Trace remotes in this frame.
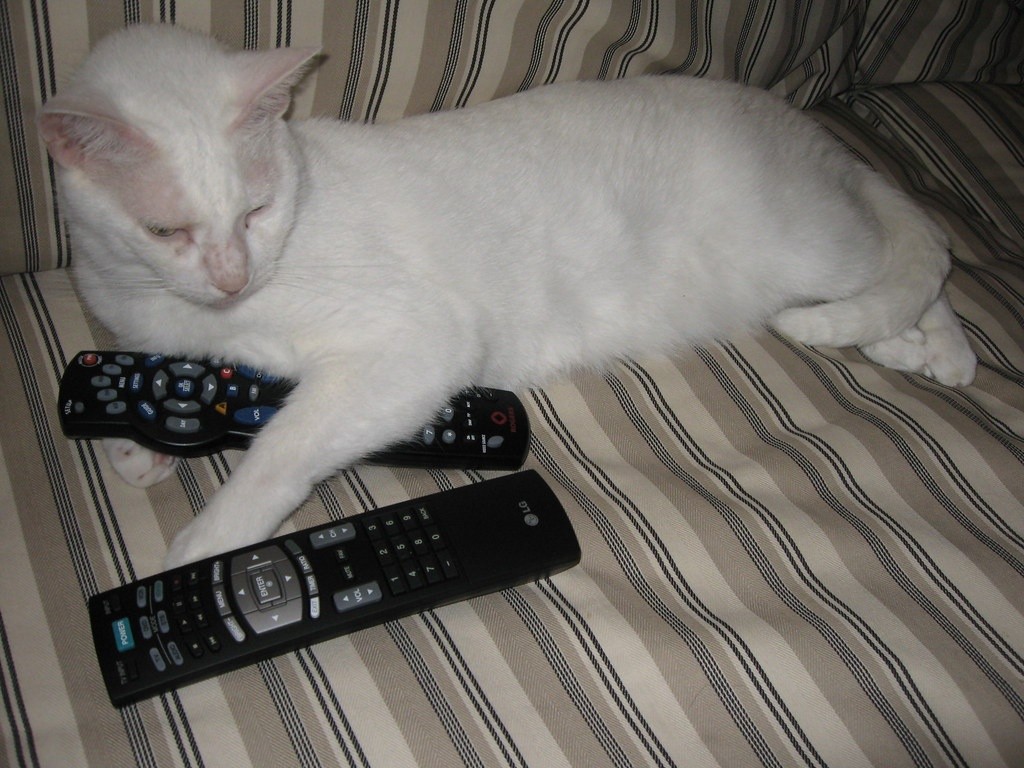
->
[55,350,532,471]
[87,469,581,708]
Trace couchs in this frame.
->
[0,0,1024,768]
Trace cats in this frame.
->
[31,15,976,569]
